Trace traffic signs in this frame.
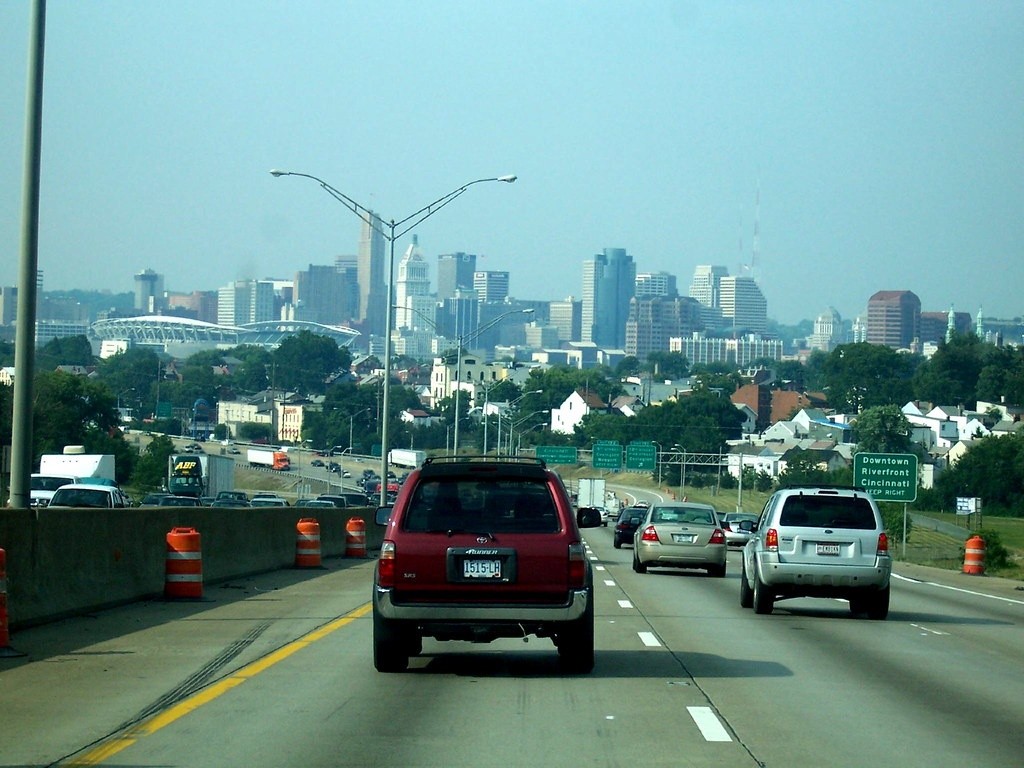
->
[593,440,657,472]
[535,446,578,464]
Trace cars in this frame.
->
[139,491,399,509]
[184,444,204,454]
[587,500,759,576]
[356,469,410,491]
[310,459,351,478]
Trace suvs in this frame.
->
[740,484,891,619]
[372,456,595,674]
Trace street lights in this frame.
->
[651,440,662,489]
[118,387,135,410]
[327,445,353,494]
[296,440,313,496]
[671,443,686,501]
[390,304,550,463]
[270,168,518,514]
[349,408,373,457]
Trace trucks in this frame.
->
[28,446,115,508]
[246,449,290,470]
[577,477,606,511]
[168,453,234,499]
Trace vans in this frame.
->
[45,484,125,509]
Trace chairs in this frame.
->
[222,495,229,500]
[237,496,243,499]
[786,510,809,526]
[417,493,454,513]
